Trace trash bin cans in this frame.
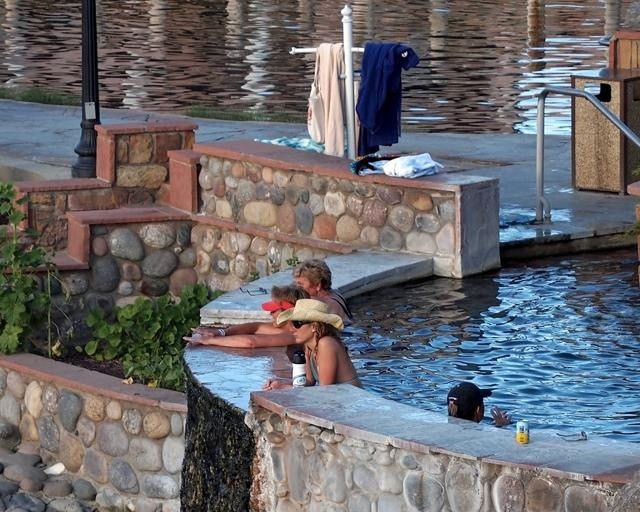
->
[570,68,640,196]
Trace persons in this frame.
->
[182,287,314,363]
[444,381,512,428]
[263,294,362,389]
[292,259,354,324]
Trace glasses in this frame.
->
[239,286,269,297]
[292,320,312,329]
[555,430,588,443]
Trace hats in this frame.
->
[447,381,493,403]
[277,298,345,333]
[261,299,295,313]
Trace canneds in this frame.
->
[517,421,529,444]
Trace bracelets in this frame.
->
[216,327,229,336]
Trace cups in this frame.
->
[292,351,309,389]
